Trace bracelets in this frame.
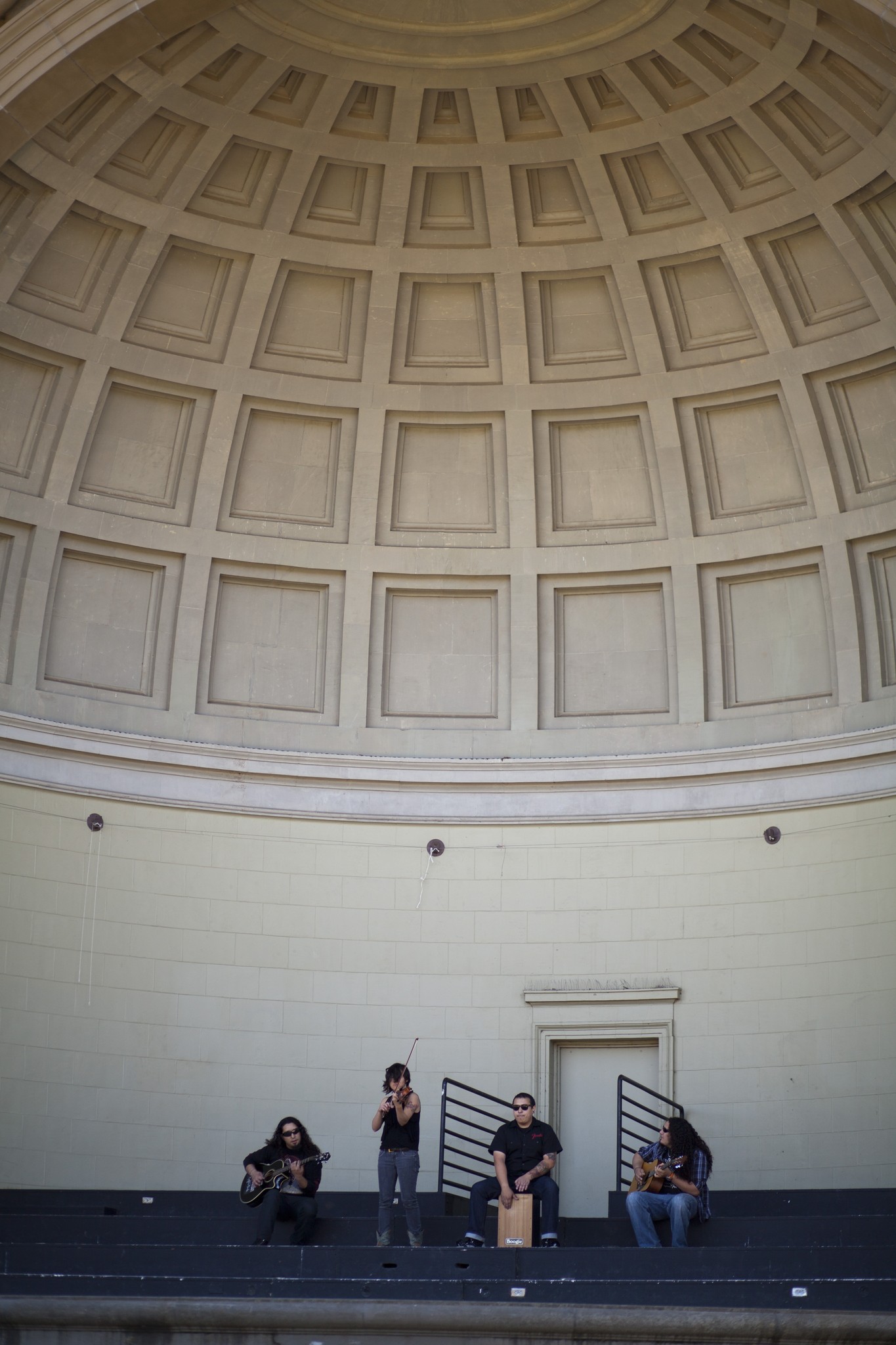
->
[634,1167,640,1174]
[250,1169,256,1177]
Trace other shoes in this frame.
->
[252,1240,269,1246]
[541,1238,560,1247]
[456,1238,483,1248]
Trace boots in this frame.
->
[376,1229,391,1246]
[407,1230,424,1246]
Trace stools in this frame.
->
[497,1193,541,1248]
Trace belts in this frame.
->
[384,1148,410,1152]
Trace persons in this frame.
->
[243,1116,322,1246]
[371,1062,425,1247]
[456,1091,563,1248]
[625,1117,713,1249]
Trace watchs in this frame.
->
[665,1172,674,1181]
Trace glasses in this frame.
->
[512,1104,532,1111]
[662,1126,668,1133]
[281,1127,301,1137]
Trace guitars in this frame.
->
[627,1155,687,1196]
[239,1151,331,1208]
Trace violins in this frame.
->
[396,1085,413,1105]
